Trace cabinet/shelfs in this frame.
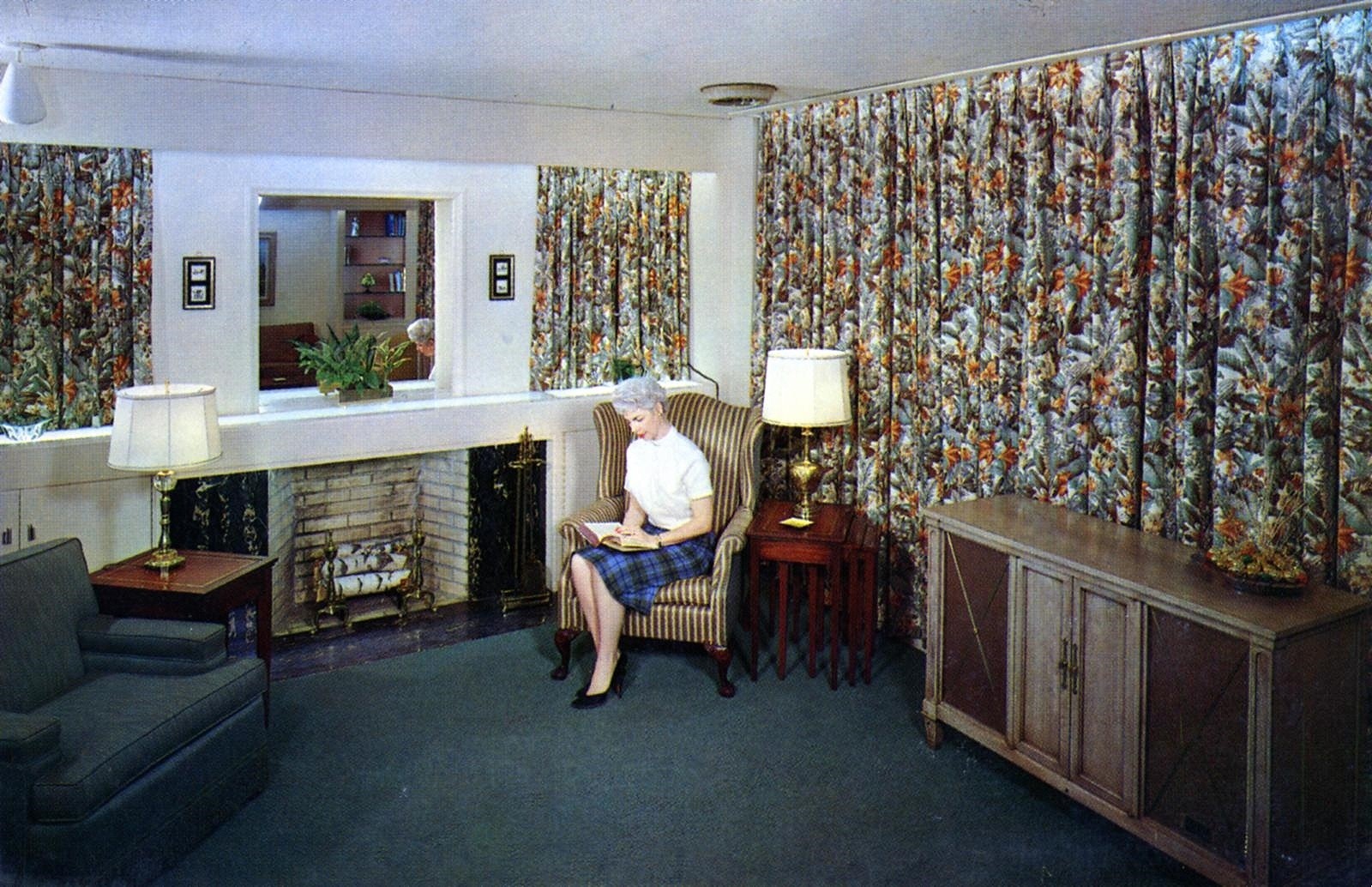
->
[915,494,1371,887]
[342,208,407,320]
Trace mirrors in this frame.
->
[255,192,435,391]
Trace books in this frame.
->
[576,520,660,552]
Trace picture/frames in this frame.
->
[259,230,275,307]
[182,257,217,310]
[489,255,515,301]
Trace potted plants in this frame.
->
[285,320,417,402]
[356,302,392,320]
[361,273,377,293]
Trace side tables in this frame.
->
[745,502,882,692]
[86,547,278,730]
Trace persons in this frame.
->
[407,318,435,380]
[564,376,716,712]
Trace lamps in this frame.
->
[109,384,224,572]
[0,41,49,127]
[701,83,777,108]
[759,347,853,519]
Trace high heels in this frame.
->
[575,651,628,694]
[569,652,623,709]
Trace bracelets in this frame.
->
[657,533,663,551]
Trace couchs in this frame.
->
[0,537,269,886]
[550,394,766,699]
[259,321,317,390]
